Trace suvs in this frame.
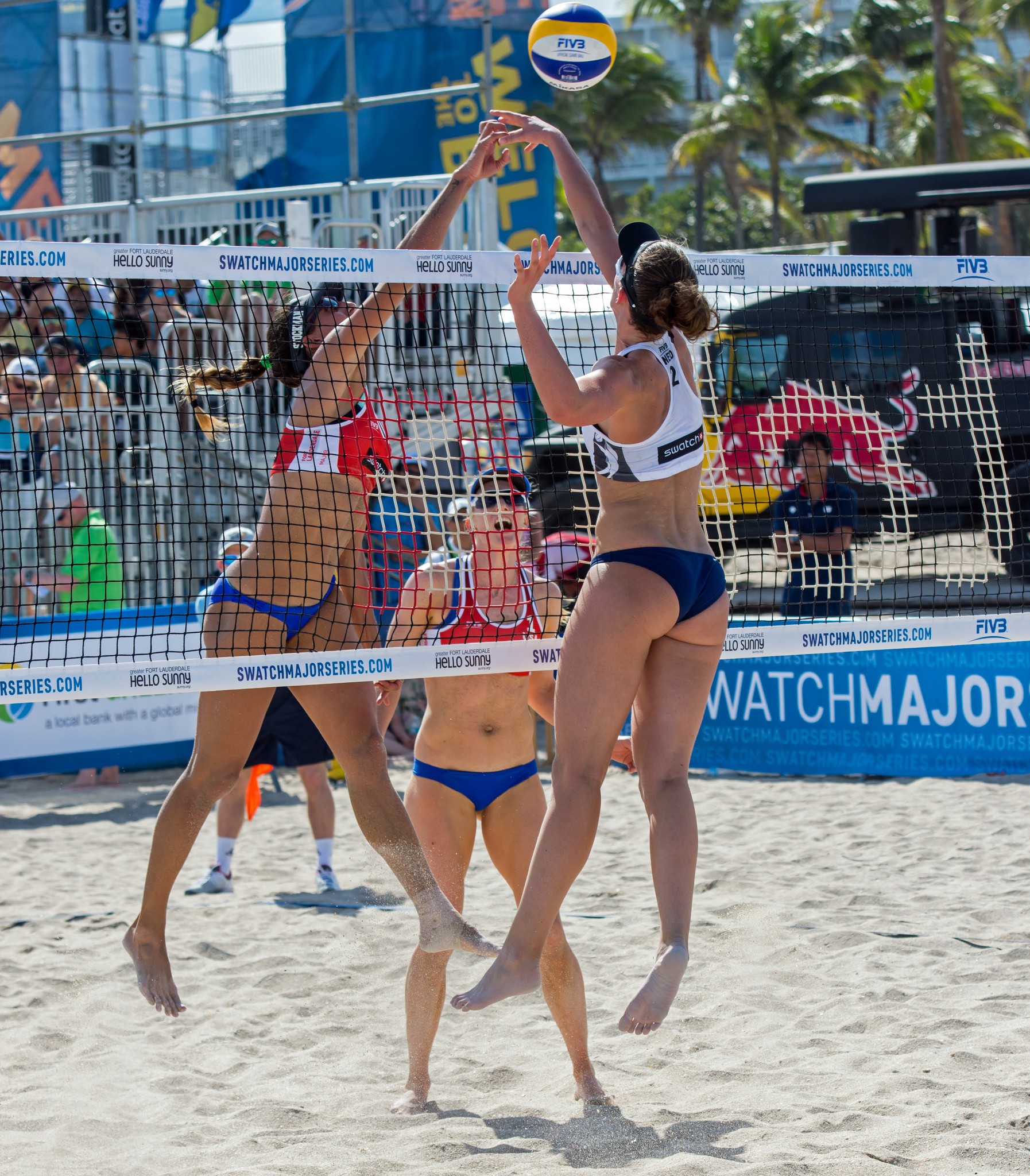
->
[521,287,1029,547]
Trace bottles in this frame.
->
[401,708,422,737]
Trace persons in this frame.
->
[771,431,859,616]
[372,465,638,1116]
[181,526,340,895]
[121,118,512,1017]
[0,224,476,787]
[448,110,731,1036]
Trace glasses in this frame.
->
[257,238,279,246]
[46,353,67,360]
[57,513,66,521]
[450,516,465,522]
[15,382,38,393]
[616,254,638,308]
[39,318,61,327]
[471,489,527,510]
[154,289,177,297]
[303,295,338,336]
[401,467,425,476]
[219,555,237,565]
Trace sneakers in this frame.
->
[315,865,343,894]
[185,863,235,894]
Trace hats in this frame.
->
[42,481,83,527]
[0,289,18,316]
[44,331,72,353]
[6,357,40,382]
[399,452,429,469]
[40,306,65,321]
[253,221,283,239]
[618,221,665,313]
[446,497,472,516]
[470,465,532,500]
[67,280,90,294]
[288,282,345,378]
[218,527,254,555]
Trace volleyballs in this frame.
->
[324,755,345,781]
[527,2,618,91]
